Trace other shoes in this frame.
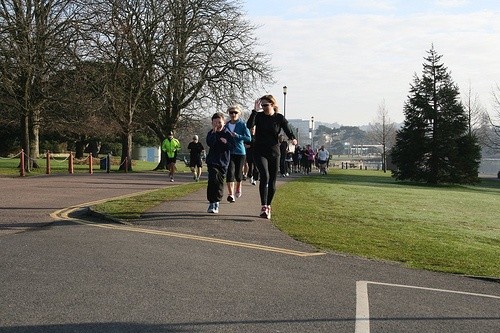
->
[193,177,199,182]
[170,177,175,182]
[242,172,260,186]
[281,170,311,178]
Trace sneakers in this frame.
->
[260,206,271,219]
[227,194,235,203]
[207,202,219,213]
[236,191,242,198]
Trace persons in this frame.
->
[205,112,235,213]
[247,95,298,219]
[244,130,330,185]
[187,135,205,182]
[224,106,251,203]
[161,131,182,182]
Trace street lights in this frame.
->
[312,117,314,150]
[283,86,287,117]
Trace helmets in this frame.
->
[166,131,173,135]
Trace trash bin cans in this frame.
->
[100,157,107,170]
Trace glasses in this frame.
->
[261,103,272,106]
[229,110,238,115]
